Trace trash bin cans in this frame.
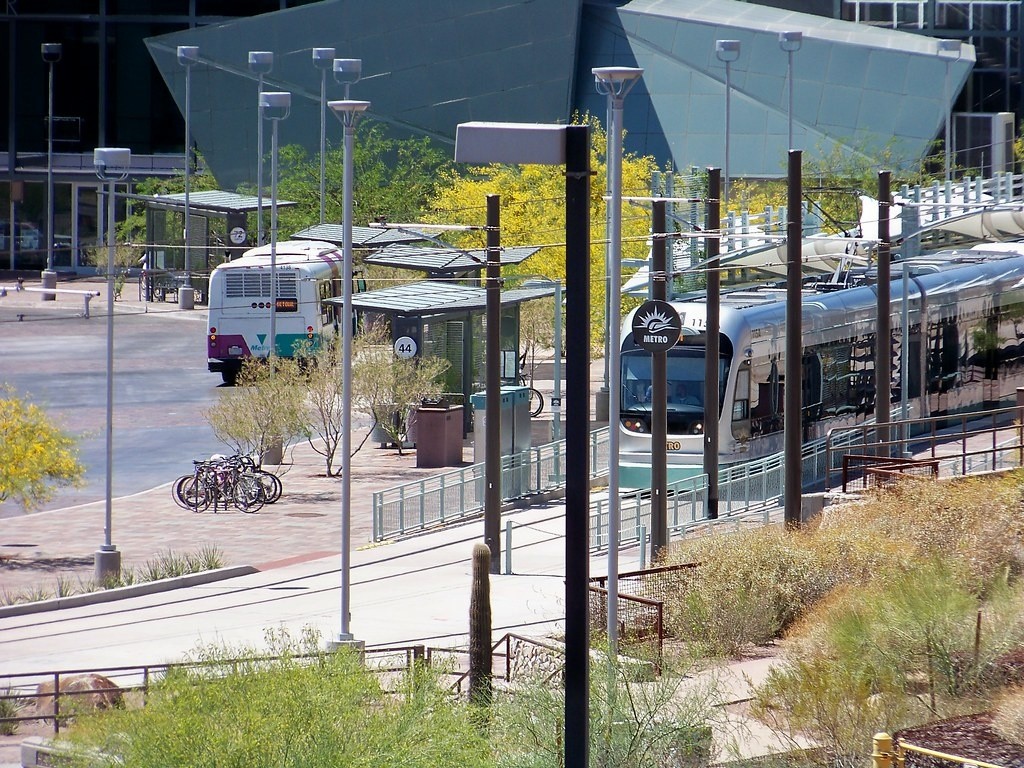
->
[370,403,418,450]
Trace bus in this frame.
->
[207,238,368,387]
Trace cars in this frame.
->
[0,222,72,253]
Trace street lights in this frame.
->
[591,67,645,713]
[712,38,746,220]
[258,91,292,389]
[775,29,804,150]
[935,38,963,209]
[248,51,275,246]
[327,97,369,676]
[312,47,336,223]
[88,146,134,587]
[330,57,364,295]
[177,45,199,311]
[39,42,63,296]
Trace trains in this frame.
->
[619,243,1024,500]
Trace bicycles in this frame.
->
[170,449,284,513]
[513,344,546,418]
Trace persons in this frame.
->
[138,243,156,274]
[670,384,700,407]
